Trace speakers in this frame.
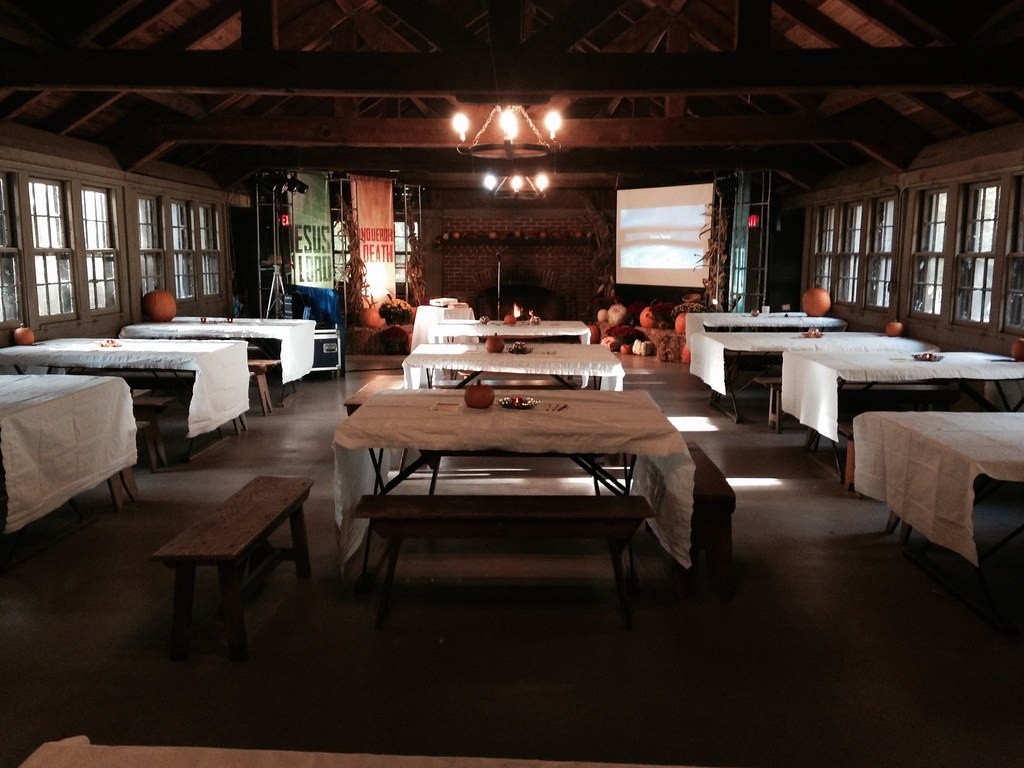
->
[284,293,308,318]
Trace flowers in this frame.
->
[379,299,412,341]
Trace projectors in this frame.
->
[429,298,458,307]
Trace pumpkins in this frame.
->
[485,333,506,353]
[800,288,831,315]
[464,379,496,408]
[358,300,382,328]
[577,303,691,362]
[14,323,35,345]
[143,286,176,321]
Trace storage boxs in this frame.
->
[312,323,341,372]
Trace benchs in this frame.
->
[153,476,314,659]
[645,441,736,573]
[350,493,657,632]
[750,376,977,541]
[343,375,404,415]
[65,358,282,510]
[419,379,607,469]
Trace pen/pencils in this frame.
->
[546,402,567,412]
[541,349,556,355]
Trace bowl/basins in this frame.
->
[681,292,702,304]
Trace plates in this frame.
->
[799,333,824,339]
[912,354,946,362]
[497,396,539,409]
[505,346,534,354]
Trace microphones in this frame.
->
[496,252,501,261]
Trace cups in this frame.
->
[761,305,770,316]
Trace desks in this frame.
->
[853,411,1024,637]
[781,350,1024,484]
[428,317,591,381]
[689,332,940,424]
[332,386,696,601]
[0,339,248,462]
[685,312,848,351]
[402,340,625,391]
[0,375,138,571]
[119,316,318,406]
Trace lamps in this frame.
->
[451,104,564,161]
[258,170,309,194]
[483,167,550,204]
